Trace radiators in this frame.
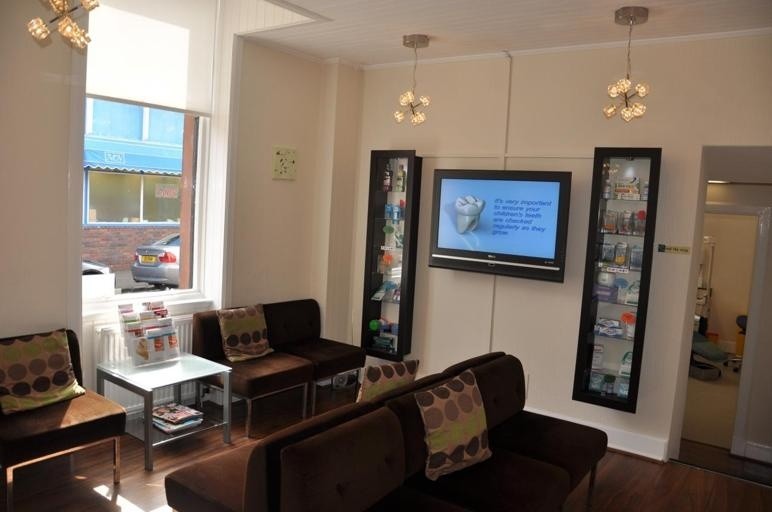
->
[102,315,202,410]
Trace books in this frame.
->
[117,300,180,366]
[142,399,204,434]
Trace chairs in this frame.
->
[263,295,364,416]
[722,314,748,373]
[192,306,312,440]
[0,330,126,505]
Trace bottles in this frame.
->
[618,351,633,394]
[602,376,616,396]
[367,163,406,337]
[597,166,648,270]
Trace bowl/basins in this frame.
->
[597,272,617,288]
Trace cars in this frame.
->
[81,259,113,275]
[132,231,180,288]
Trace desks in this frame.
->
[95,353,233,472]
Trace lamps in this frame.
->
[394,34,430,126]
[24,0,100,50]
[602,6,649,123]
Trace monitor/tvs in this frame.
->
[427,168,573,284]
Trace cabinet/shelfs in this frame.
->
[361,149,422,362]
[572,147,662,414]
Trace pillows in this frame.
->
[0,327,86,415]
[216,305,274,363]
